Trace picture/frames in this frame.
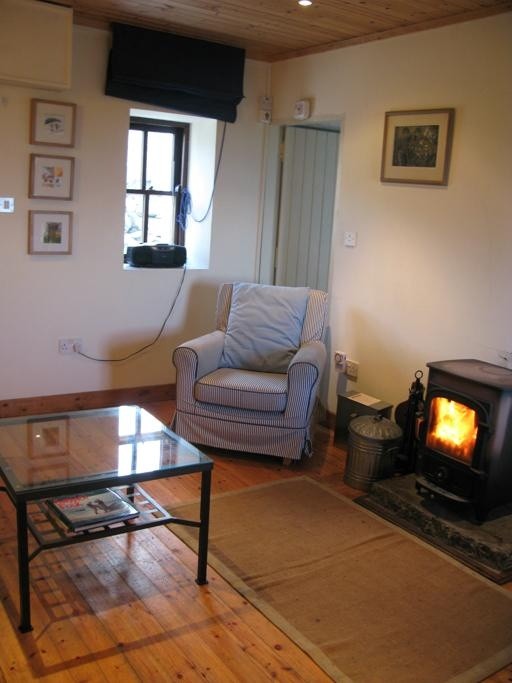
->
[27,208,75,257]
[28,462,70,486]
[29,97,77,148]
[28,153,76,200]
[378,105,458,188]
[26,414,69,458]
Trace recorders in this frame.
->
[127,244,186,269]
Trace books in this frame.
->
[45,488,140,533]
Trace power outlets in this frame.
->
[343,230,360,248]
[58,338,83,355]
[345,358,360,378]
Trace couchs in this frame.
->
[168,280,331,468]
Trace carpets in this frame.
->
[153,475,512,683]
